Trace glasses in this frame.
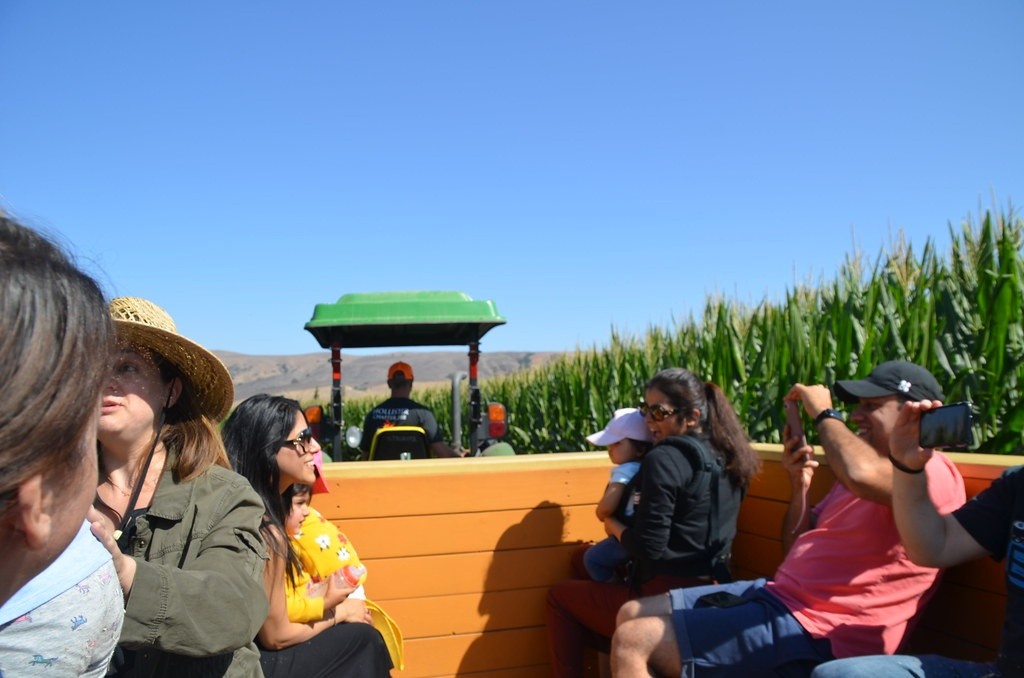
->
[639,402,691,421]
[281,426,312,454]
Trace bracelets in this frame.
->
[888,450,924,475]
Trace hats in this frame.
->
[585,408,653,446]
[105,296,234,426]
[833,360,945,405]
[388,361,413,381]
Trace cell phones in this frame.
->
[783,398,810,463]
[918,401,972,450]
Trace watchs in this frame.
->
[815,408,844,426]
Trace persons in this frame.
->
[812,400,1024,678]
[0,518,124,678]
[219,393,395,678]
[280,483,357,623]
[88,298,271,678]
[584,367,763,590]
[0,217,114,607]
[611,360,966,678]
[360,361,462,462]
